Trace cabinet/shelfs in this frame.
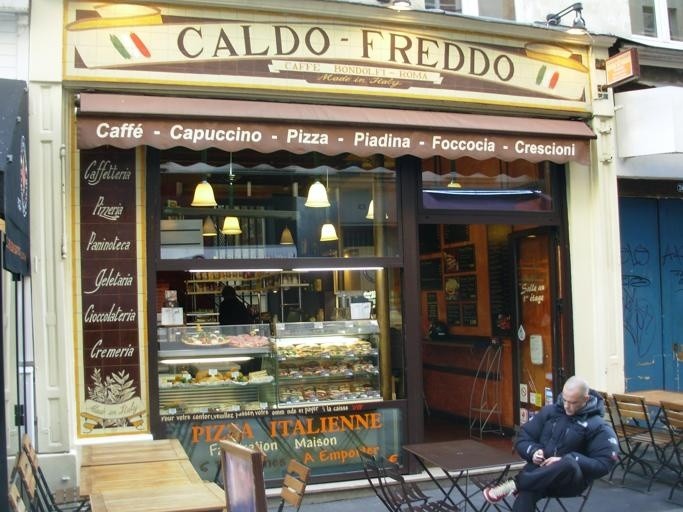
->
[184,272,311,329]
[156,318,384,415]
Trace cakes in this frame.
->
[157,333,269,412]
[275,339,374,401]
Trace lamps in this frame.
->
[318,169,338,242]
[279,222,294,245]
[365,198,388,222]
[374,0,412,9]
[222,215,242,235]
[544,0,586,35]
[304,178,332,209]
[200,216,218,236]
[189,179,219,207]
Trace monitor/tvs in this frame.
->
[350,301,373,319]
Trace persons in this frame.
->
[483,375,621,511]
[218,285,253,335]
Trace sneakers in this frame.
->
[483,480,516,504]
